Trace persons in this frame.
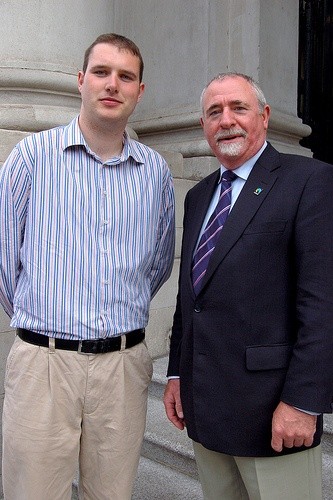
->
[162,72,332,499]
[0,34,176,500]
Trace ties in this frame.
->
[191,171,238,301]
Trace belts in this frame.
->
[15,328,145,354]
